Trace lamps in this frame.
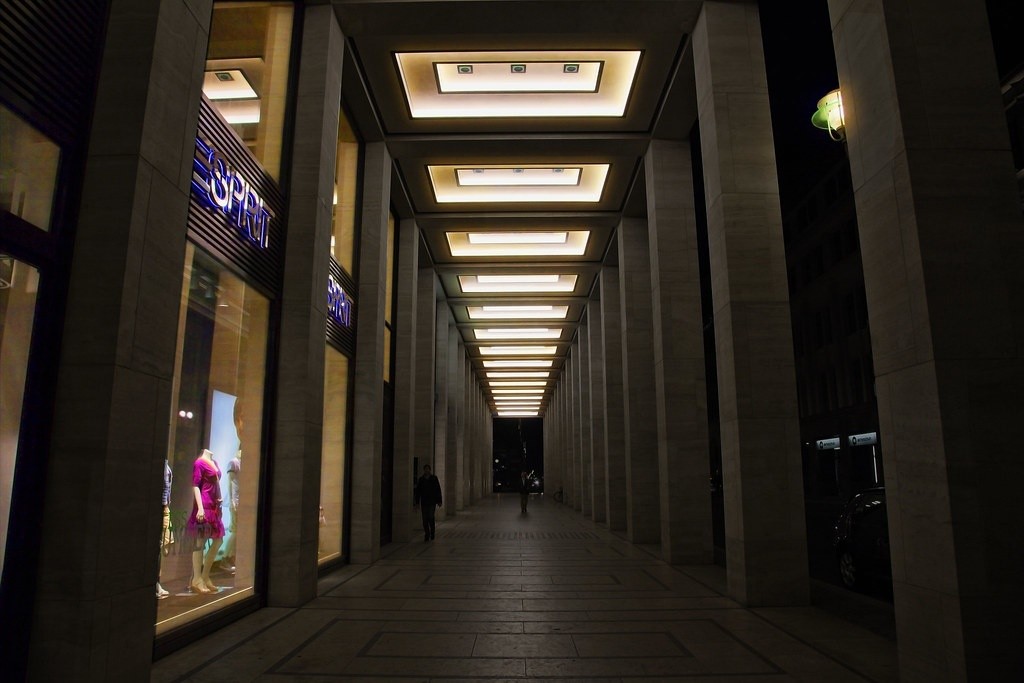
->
[811,86,845,142]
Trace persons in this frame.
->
[521,472,530,513]
[219,450,241,572]
[187,448,226,594]
[412,464,442,543]
[156,460,174,598]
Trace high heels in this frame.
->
[207,585,218,590]
[191,581,210,594]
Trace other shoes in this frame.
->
[424,534,429,542]
[431,530,435,540]
[156,582,170,597]
